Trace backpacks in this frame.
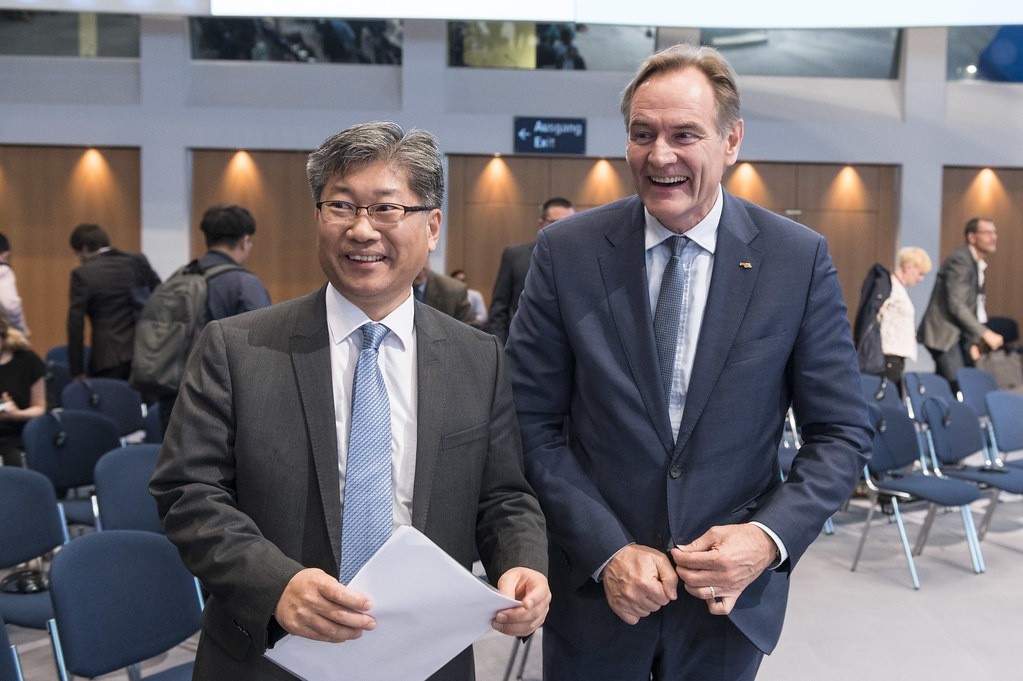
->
[129,259,246,397]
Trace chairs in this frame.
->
[0,346,206,681]
[779,366,1022,592]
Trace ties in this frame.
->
[416,291,424,303]
[653,236,690,411]
[339,323,392,586]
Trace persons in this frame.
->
[133,203,272,441]
[412,197,575,347]
[0,231,48,467]
[916,217,1004,398]
[852,247,932,404]
[66,223,163,381]
[148,121,552,681]
[503,44,875,681]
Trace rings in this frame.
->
[710,586,715,599]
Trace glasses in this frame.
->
[976,230,997,235]
[316,200,441,226]
